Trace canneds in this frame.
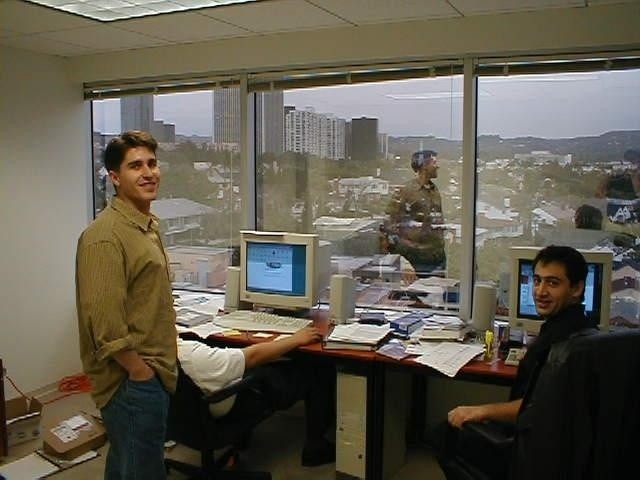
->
[493,321,511,359]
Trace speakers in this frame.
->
[224,266,252,312]
[328,274,356,324]
[472,282,496,339]
[380,254,400,290]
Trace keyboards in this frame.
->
[213,310,314,334]
[503,347,528,366]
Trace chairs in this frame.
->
[164,355,272,480]
[436,324,639,479]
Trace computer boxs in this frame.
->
[335,364,383,480]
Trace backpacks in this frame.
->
[606,176,639,222]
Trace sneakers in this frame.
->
[301,438,336,466]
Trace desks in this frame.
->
[182,310,523,445]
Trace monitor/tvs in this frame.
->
[508,246,613,338]
[313,216,379,292]
[240,232,331,318]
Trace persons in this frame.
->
[596,149,640,236]
[175,328,337,466]
[435,246,601,478]
[575,204,601,229]
[382,150,447,278]
[380,227,420,284]
[75,131,179,480]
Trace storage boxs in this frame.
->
[4,375,107,461]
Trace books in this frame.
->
[175,308,213,328]
[408,273,464,308]
[0,447,101,480]
[323,309,483,376]
